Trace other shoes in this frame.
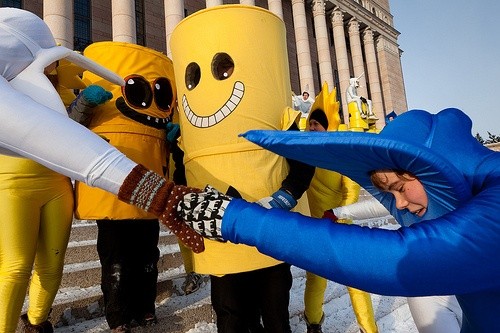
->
[140,311,158,326]
[111,323,130,333]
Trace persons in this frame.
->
[178,108,500,333]
[0,7,207,253]
[1,3,379,333]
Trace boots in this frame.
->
[303,308,324,333]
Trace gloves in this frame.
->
[177,183,233,243]
[118,164,205,254]
[256,190,297,211]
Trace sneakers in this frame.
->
[183,272,203,296]
